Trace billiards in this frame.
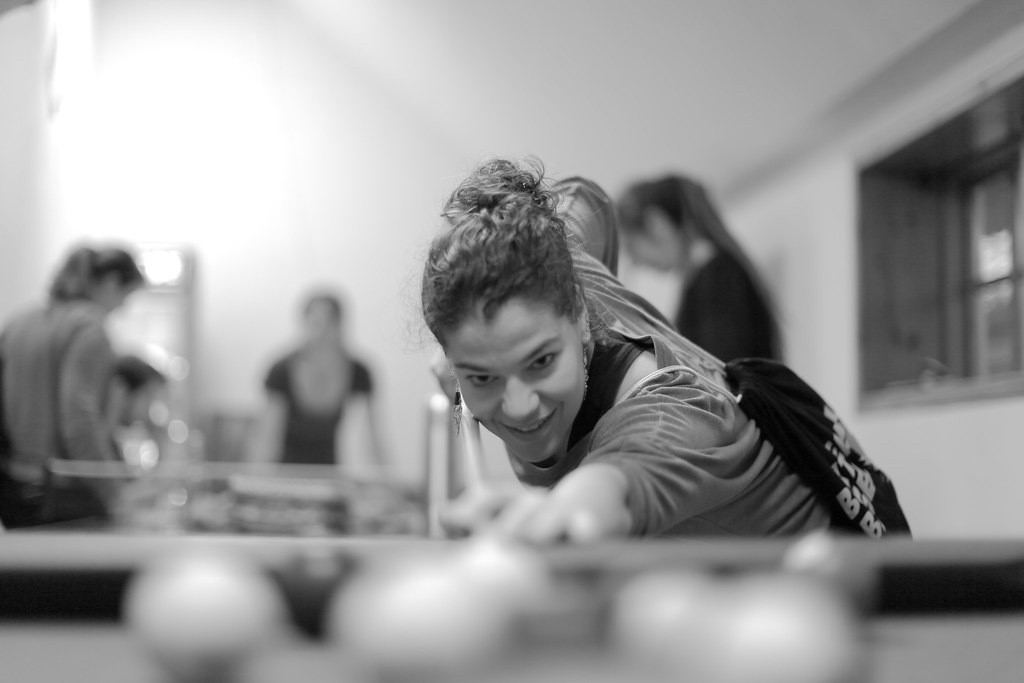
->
[121,531,887,682]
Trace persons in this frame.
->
[0,248,167,622]
[617,175,783,359]
[424,160,831,541]
[257,294,375,465]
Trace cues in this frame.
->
[455,385,499,539]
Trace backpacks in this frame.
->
[615,302,914,541]
